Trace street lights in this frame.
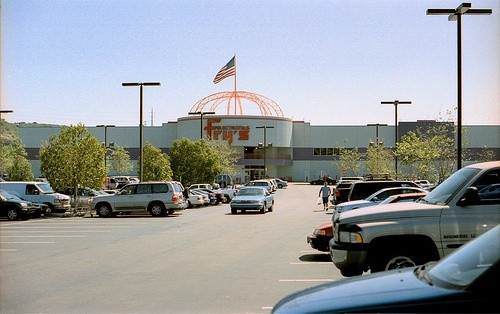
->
[255,126,274,179]
[367,123,388,176]
[96,124,116,175]
[188,112,216,140]
[381,100,412,180]
[426,2,493,170]
[122,82,161,183]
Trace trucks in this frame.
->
[0,181,71,214]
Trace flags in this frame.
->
[212,56,235,84]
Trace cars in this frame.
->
[259,178,288,192]
[306,192,428,253]
[309,177,338,185]
[230,186,274,214]
[58,176,140,209]
[0,189,51,222]
[331,186,430,222]
[186,183,244,209]
[329,177,437,205]
[270,222,500,314]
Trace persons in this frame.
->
[319,182,331,211]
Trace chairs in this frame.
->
[134,186,138,194]
[257,190,262,194]
[28,186,33,194]
[242,190,247,194]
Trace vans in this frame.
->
[348,180,423,201]
[243,180,273,194]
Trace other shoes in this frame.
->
[326,208,328,211]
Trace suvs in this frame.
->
[90,180,187,218]
[329,161,500,277]
